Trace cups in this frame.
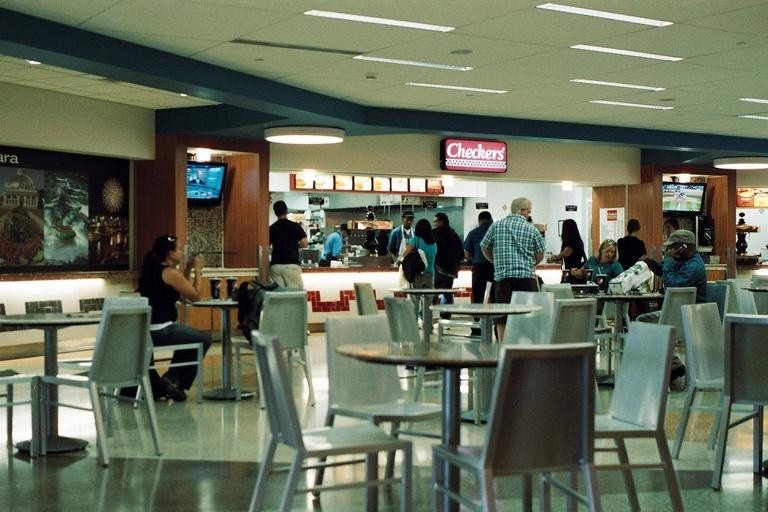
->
[596,274,607,292]
[585,269,592,282]
[225,278,239,300]
[208,277,221,301]
[561,269,570,283]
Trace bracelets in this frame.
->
[193,270,202,275]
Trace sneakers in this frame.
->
[159,375,187,401]
[671,364,684,379]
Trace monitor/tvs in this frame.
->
[186,161,229,207]
[661,181,709,216]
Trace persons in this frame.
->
[387,210,465,331]
[319,223,352,268]
[268,199,312,336]
[134,234,213,402]
[189,170,205,185]
[480,198,707,381]
[462,212,498,337]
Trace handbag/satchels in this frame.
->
[609,260,654,295]
[403,250,425,282]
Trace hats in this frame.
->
[665,230,695,245]
[402,211,415,218]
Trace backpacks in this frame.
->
[237,279,281,346]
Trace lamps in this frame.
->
[264,127,346,145]
[714,157,768,169]
